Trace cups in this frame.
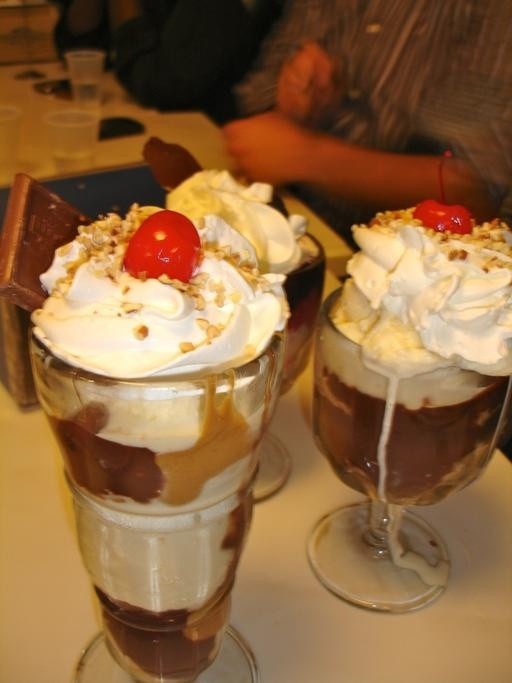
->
[64,49,105,114]
[44,108,101,174]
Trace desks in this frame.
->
[1,1,512,683]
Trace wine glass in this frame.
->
[28,324,259,681]
[252,232,330,504]
[306,287,512,619]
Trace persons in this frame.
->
[52,0,283,113]
[221,0,512,254]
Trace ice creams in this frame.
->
[0,172,290,683]
[311,150,512,507]
[143,136,325,398]
